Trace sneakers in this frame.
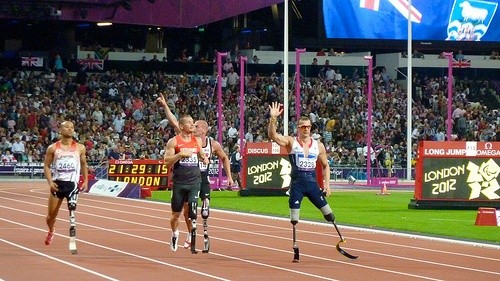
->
[184,234,192,249]
[170,231,180,253]
[45,230,55,246]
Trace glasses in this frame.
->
[298,125,312,130]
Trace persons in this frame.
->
[44,119,89,245]
[0,39,500,191]
[164,115,210,251]
[268,102,335,223]
[158,92,234,249]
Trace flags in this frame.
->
[20,56,44,68]
[323,0,500,41]
[76,58,105,72]
[453,59,471,68]
